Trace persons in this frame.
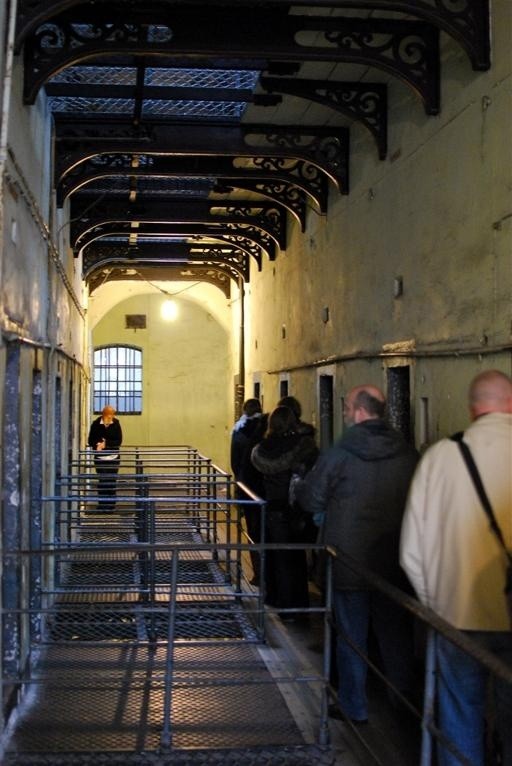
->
[291,381,422,727]
[396,366,512,765]
[229,397,322,621]
[87,404,123,516]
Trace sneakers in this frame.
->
[249,577,299,623]
[327,702,368,722]
[387,684,414,711]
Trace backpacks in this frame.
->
[236,416,264,486]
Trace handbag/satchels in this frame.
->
[289,509,322,540]
[500,546,512,625]
